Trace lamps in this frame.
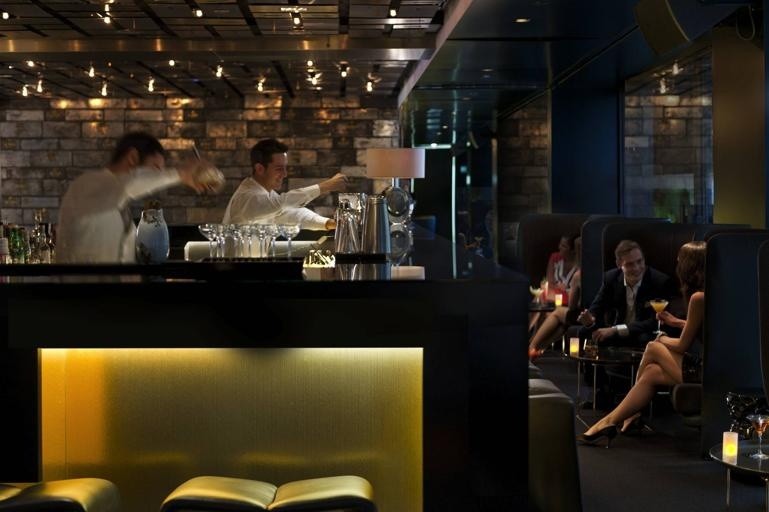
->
[366,147,425,225]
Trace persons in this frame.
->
[528,229,581,342]
[526,238,581,364]
[221,139,348,258]
[55,132,218,283]
[577,240,675,409]
[576,241,706,449]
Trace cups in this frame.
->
[554,293,563,308]
[570,338,579,355]
[722,431,738,465]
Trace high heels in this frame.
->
[575,424,617,450]
[529,348,546,361]
[618,414,644,438]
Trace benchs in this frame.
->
[159,475,379,512]
[0,477,122,512]
[528,379,583,512]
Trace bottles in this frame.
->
[6,222,56,264]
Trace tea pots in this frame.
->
[334,196,393,255]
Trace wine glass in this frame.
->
[194,222,303,259]
[530,287,544,308]
[649,298,669,337]
[746,413,769,462]
